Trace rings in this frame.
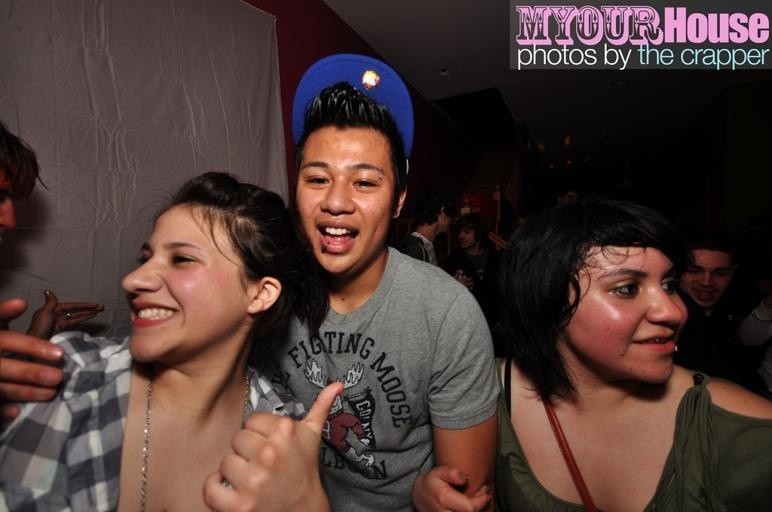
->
[64,310,72,321]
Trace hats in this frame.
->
[291,52,416,175]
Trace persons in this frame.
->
[0,169,345,512]
[411,195,770,512]
[253,79,501,510]
[383,183,770,401]
[0,124,104,424]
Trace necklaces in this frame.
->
[138,368,251,511]
